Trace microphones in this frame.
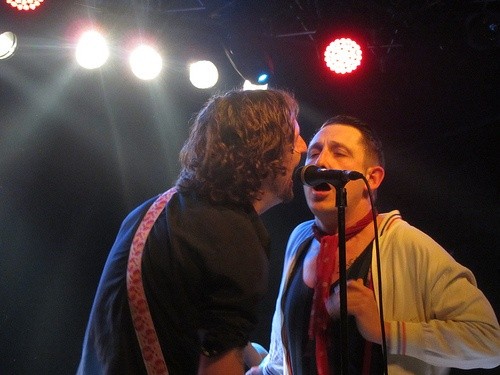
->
[296,164,364,187]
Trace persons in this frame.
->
[246,115,500,375]
[75,90,307,375]
